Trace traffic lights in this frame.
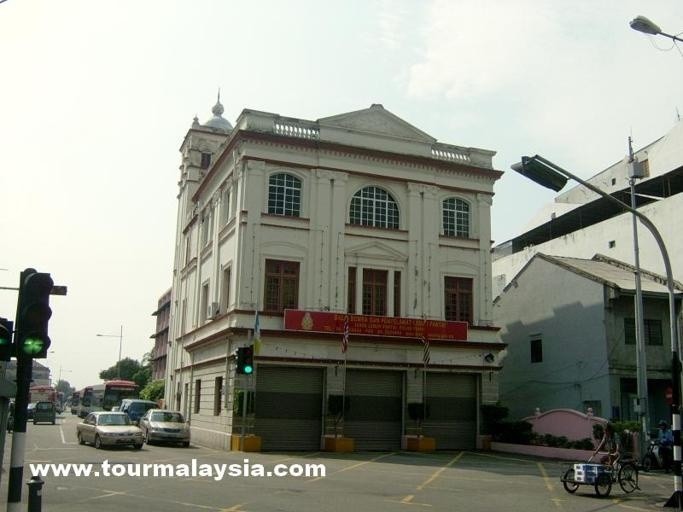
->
[235,344,253,375]
[18,267,55,359]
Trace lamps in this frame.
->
[485,354,494,363]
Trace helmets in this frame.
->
[658,419,668,426]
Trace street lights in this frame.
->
[509,152,683,509]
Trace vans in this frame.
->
[33,400,56,425]
[120,398,160,425]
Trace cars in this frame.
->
[76,411,144,449]
[139,408,191,448]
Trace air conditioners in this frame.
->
[206,302,219,319]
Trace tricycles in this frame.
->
[560,442,642,498]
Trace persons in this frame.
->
[594,427,626,482]
[658,420,674,446]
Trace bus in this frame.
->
[80,378,140,418]
